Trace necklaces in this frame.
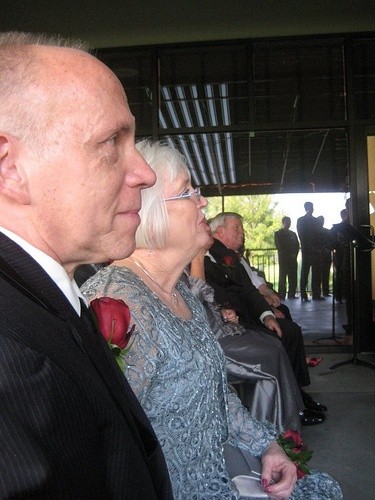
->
[130,253,182,310]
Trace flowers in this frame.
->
[276,427,314,479]
[88,297,140,373]
[222,256,236,270]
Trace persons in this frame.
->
[316,215,332,297]
[0,31,175,500]
[179,247,305,433]
[204,211,330,427]
[296,201,326,303]
[328,209,349,304]
[80,139,343,500]
[236,235,293,319]
[273,215,301,301]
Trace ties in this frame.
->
[78,297,100,343]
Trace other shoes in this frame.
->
[343,324,352,335]
[281,296,284,300]
[288,296,299,299]
[304,354,321,367]
[298,408,325,426]
[324,295,331,297]
[302,395,327,412]
[301,296,308,303]
[313,297,325,300]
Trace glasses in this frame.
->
[160,187,201,203]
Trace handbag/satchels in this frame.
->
[223,444,269,500]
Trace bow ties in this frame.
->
[229,248,241,256]
